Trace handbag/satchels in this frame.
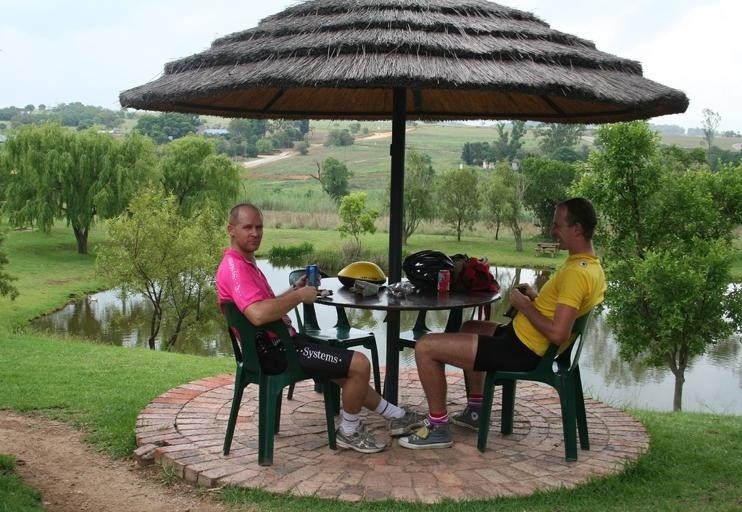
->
[450,254,501,293]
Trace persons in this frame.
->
[396,197,605,448]
[214,201,427,454]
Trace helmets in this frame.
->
[402,249,455,293]
[336,261,387,288]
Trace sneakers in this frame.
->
[452,405,481,433]
[398,420,454,450]
[390,410,426,437]
[335,422,389,455]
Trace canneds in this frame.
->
[307,265,319,285]
[437,269,451,292]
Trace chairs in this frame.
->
[399,306,591,462]
[223,268,383,467]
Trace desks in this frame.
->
[317,277,503,405]
